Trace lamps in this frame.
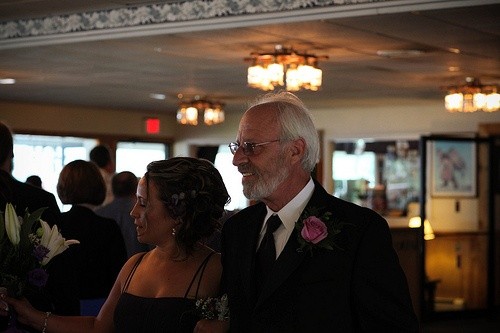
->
[444,76,500,113]
[176,92,225,125]
[242,45,330,91]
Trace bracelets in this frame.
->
[41,312,51,333]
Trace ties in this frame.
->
[257,215,282,268]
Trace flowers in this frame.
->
[194,294,230,322]
[0,202,81,314]
[295,210,334,253]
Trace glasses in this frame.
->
[230,138,281,155]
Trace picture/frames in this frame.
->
[432,142,479,198]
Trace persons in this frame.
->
[39,160,127,317]
[26,176,42,189]
[0,121,79,316]
[93,171,155,276]
[90,145,117,213]
[217,90,422,333]
[0,155,231,333]
